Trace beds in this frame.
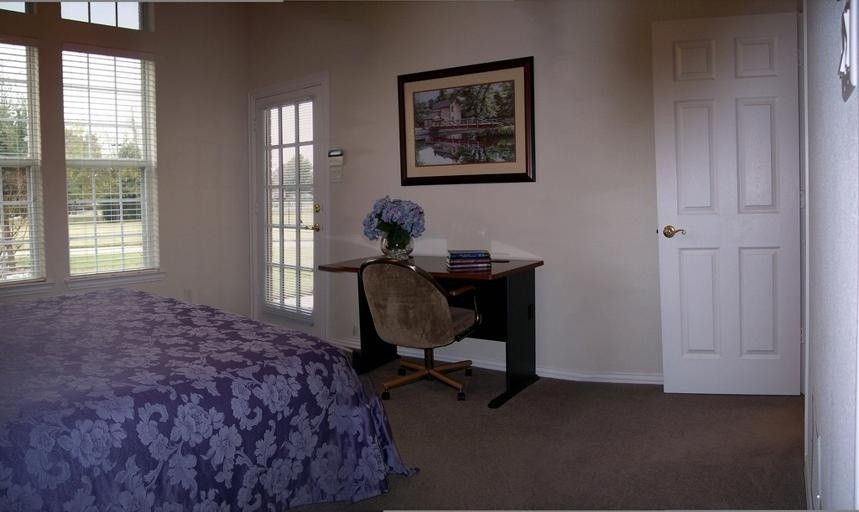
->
[0,288,420,512]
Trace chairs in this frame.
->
[357,259,486,402]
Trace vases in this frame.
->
[380,232,416,260]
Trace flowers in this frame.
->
[361,193,426,260]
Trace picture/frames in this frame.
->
[397,55,536,187]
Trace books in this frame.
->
[446,250,492,273]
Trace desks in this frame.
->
[318,251,545,409]
